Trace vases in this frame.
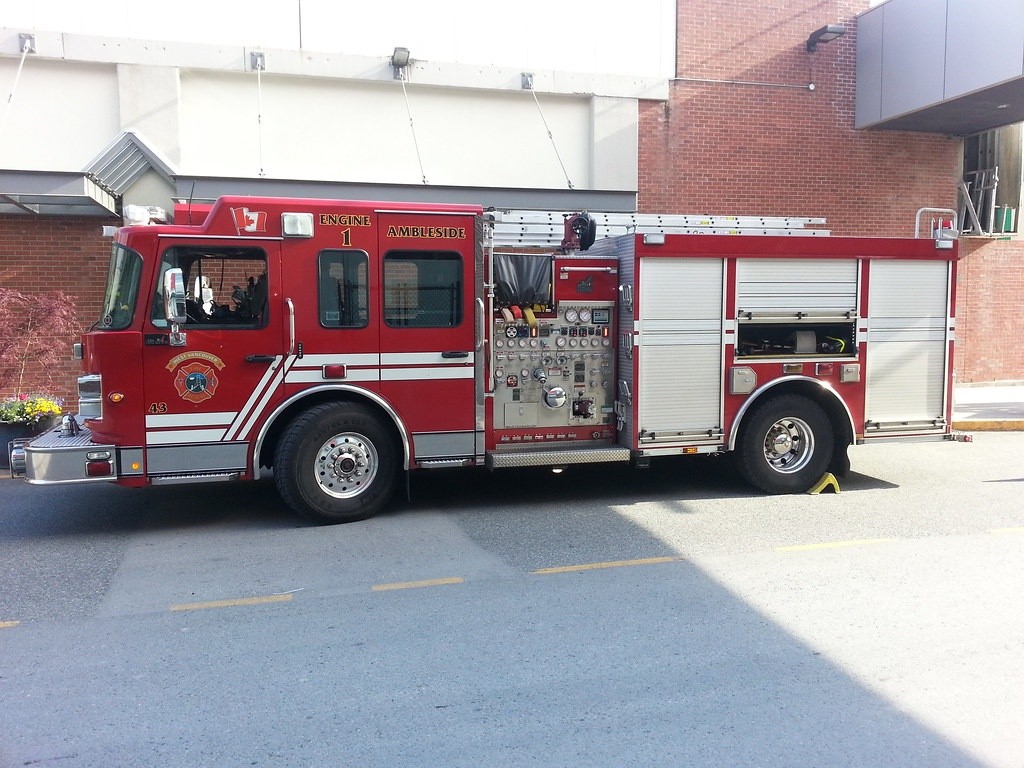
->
[1,416,56,469]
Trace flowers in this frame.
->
[0,394,65,430]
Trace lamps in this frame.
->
[805,22,846,51]
[391,47,410,67]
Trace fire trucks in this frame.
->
[8,195,972,525]
